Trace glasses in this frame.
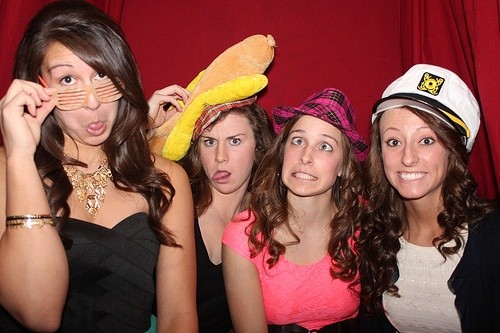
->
[37,74,127,111]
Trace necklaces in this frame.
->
[62,152,112,220]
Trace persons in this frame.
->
[354,64,500,333]
[146,84,276,333]
[219,88,369,333]
[0,0,198,333]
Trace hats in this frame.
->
[371,63,481,155]
[270,88,370,167]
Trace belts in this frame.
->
[267,318,361,333]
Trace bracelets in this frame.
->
[6,215,56,229]
